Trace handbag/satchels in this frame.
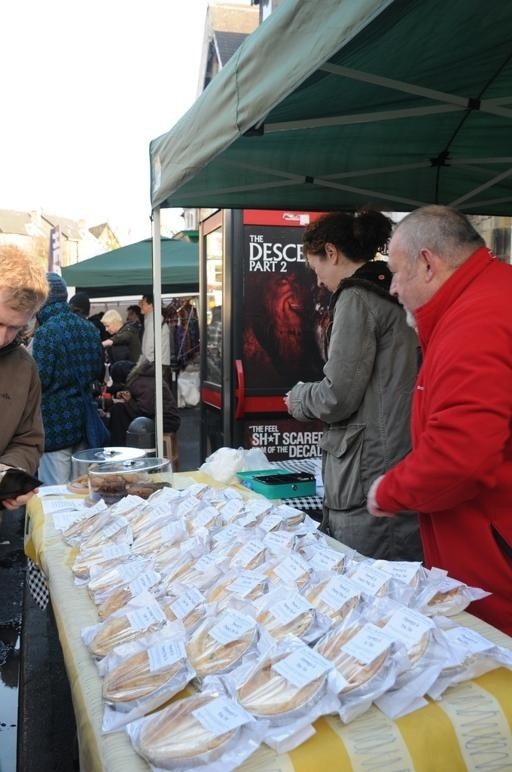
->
[83,394,112,448]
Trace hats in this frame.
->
[44,272,68,305]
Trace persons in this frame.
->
[0,244,200,520]
[263,201,427,567]
[362,206,510,646]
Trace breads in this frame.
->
[63,494,482,760]
